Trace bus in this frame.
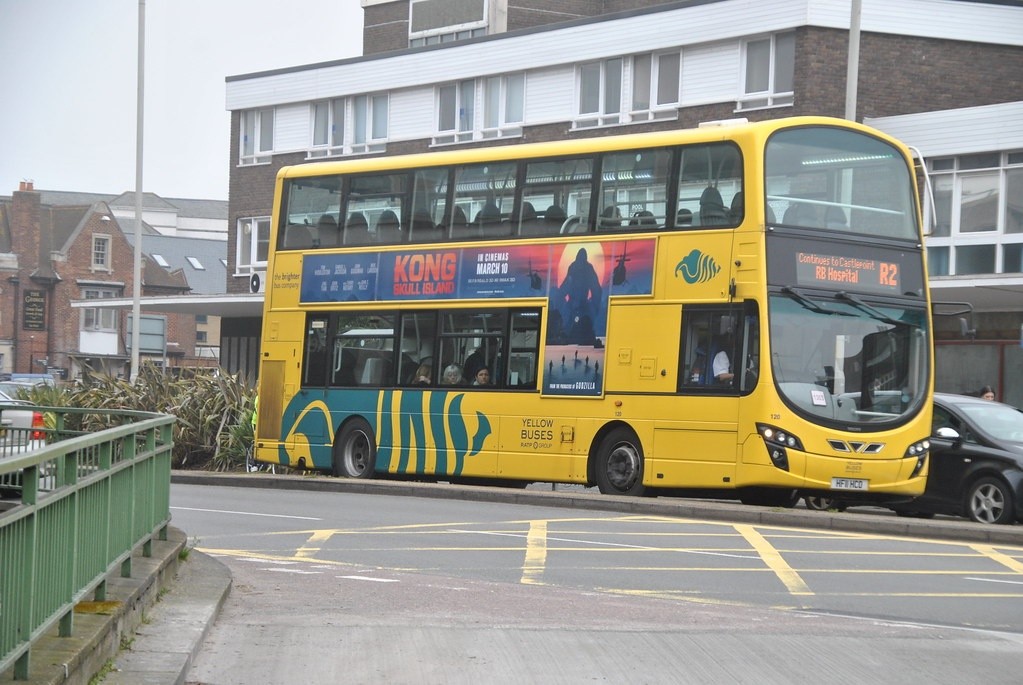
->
[127,365,219,389]
[250,115,977,510]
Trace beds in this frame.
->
[317,214,337,245]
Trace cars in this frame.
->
[0,371,58,498]
[800,390,1023,528]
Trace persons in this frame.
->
[465,337,498,378]
[412,358,433,385]
[473,367,492,385]
[250,384,260,472]
[442,366,465,384]
[980,386,994,400]
[711,337,754,386]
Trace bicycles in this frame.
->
[245,439,295,475]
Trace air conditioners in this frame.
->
[249,270,267,293]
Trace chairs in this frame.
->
[795,204,819,227]
[825,206,850,231]
[629,210,657,225]
[415,208,433,242]
[348,212,368,242]
[285,223,313,248]
[601,206,621,226]
[543,205,567,236]
[731,191,776,223]
[699,187,724,226]
[474,205,511,236]
[678,209,692,224]
[522,202,537,236]
[781,205,795,225]
[376,211,399,243]
[359,357,382,384]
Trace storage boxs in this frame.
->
[441,206,466,238]
